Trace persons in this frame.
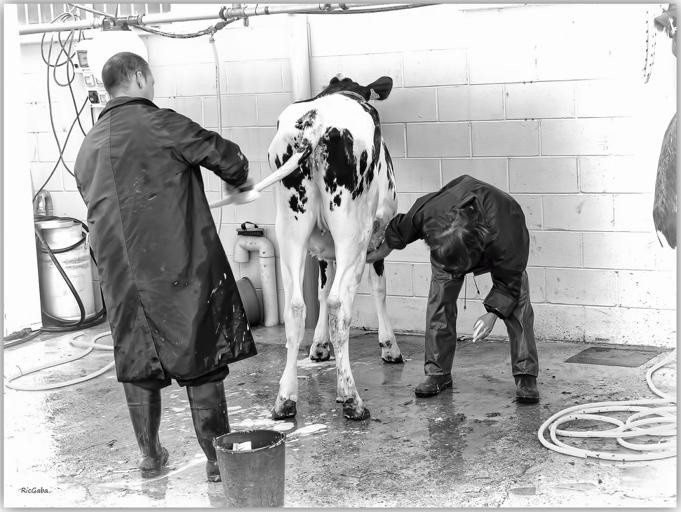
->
[367,174,540,403]
[75,49,249,483]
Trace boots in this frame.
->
[124,382,168,479]
[186,382,232,483]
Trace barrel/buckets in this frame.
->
[212,428,286,509]
[235,275,262,327]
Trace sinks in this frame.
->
[34,220,83,250]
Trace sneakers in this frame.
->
[514,374,540,402]
[415,373,453,398]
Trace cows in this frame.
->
[208,76,404,421]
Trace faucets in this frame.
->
[33,189,53,216]
